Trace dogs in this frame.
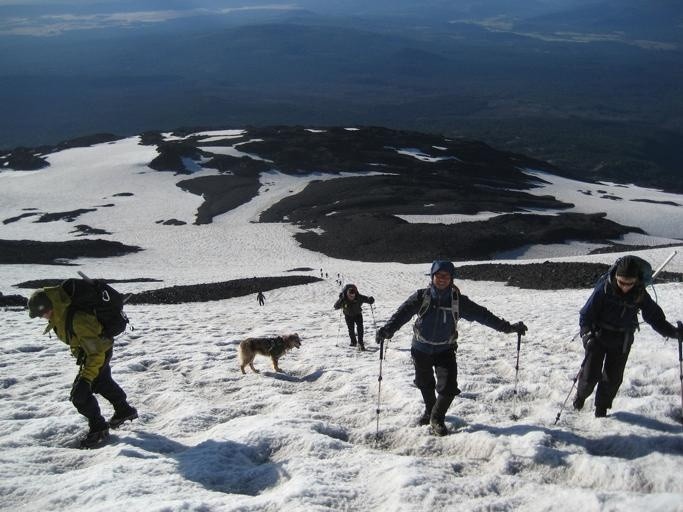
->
[240,333,301,374]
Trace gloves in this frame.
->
[375,327,389,344]
[669,324,682,338]
[581,331,599,352]
[367,297,374,304]
[510,322,527,336]
[71,380,92,404]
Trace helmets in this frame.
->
[26,290,52,319]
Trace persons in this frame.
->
[376,253,528,437]
[573,254,682,417]
[26,278,139,449]
[256,291,266,305]
[332,283,375,351]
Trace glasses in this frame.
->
[616,279,635,286]
[434,272,451,280]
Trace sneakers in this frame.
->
[107,407,137,429]
[350,341,356,346]
[414,414,430,424]
[594,407,606,417]
[357,344,364,351]
[430,419,447,437]
[572,392,584,409]
[80,419,109,450]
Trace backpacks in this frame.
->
[61,278,128,345]
